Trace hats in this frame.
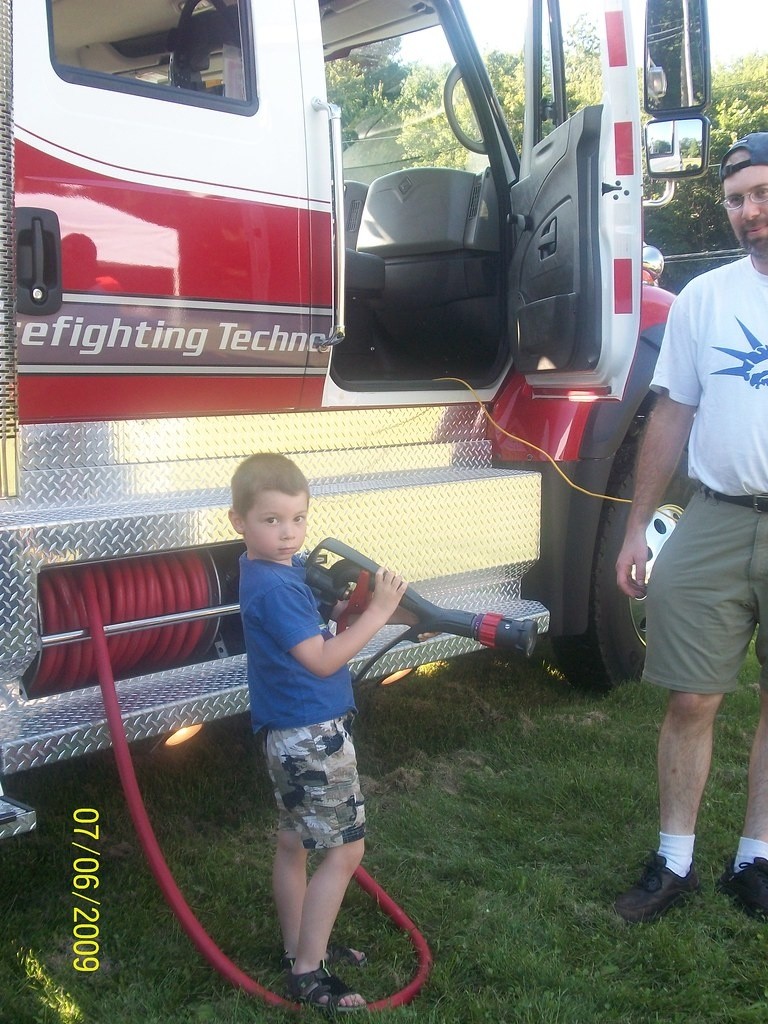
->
[718,131,768,183]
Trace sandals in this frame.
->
[283,961,369,1011]
[273,941,366,974]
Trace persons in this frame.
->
[614,132,768,925]
[229,454,409,1013]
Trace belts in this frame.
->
[701,483,768,514]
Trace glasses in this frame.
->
[722,187,768,210]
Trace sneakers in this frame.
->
[612,850,700,927]
[715,857,768,924]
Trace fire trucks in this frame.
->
[1,0,715,843]
